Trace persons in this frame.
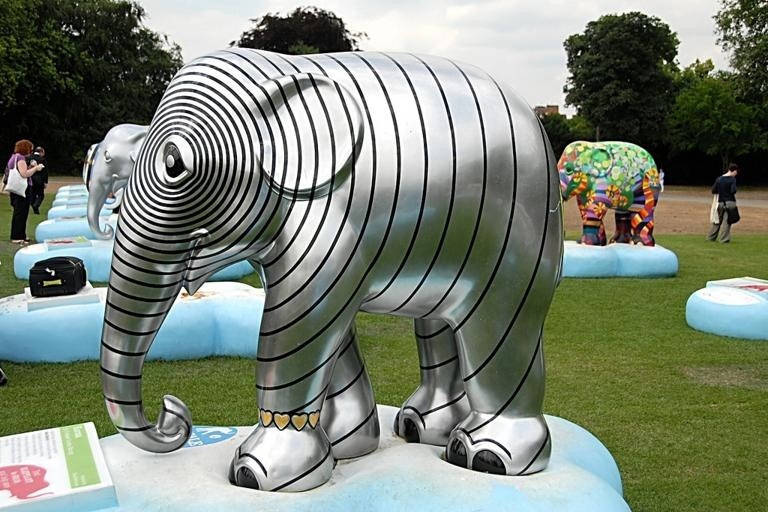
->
[7,140,45,244]
[705,164,738,244]
[27,147,48,214]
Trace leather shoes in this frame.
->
[32,203,40,215]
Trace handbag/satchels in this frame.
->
[5,157,27,198]
[728,207,740,224]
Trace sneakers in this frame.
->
[12,240,30,244]
[25,237,31,241]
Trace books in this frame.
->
[0,419,118,512]
